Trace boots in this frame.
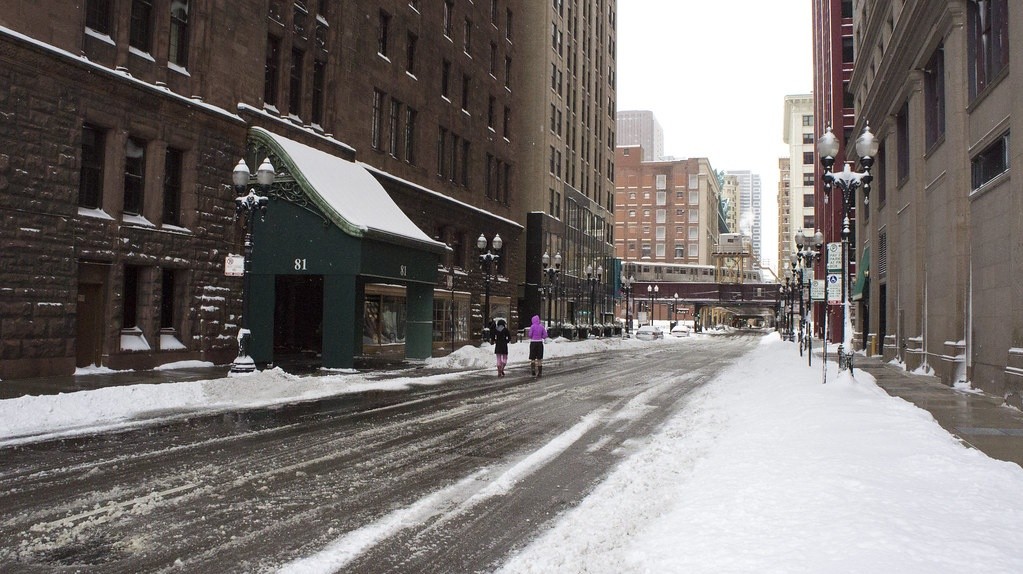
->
[497,366,501,377]
[500,363,505,376]
[537,366,543,377]
[531,363,536,376]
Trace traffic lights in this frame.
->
[671,303,674,313]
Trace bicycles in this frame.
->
[842,350,854,377]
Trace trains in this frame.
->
[619,260,761,285]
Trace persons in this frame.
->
[492,320,511,377]
[489,318,496,344]
[528,316,547,376]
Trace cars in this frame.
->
[671,325,690,338]
[636,326,664,341]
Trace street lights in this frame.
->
[815,120,881,380]
[229,158,276,377]
[647,285,659,326]
[620,276,634,338]
[674,292,678,321]
[586,264,603,340]
[542,251,563,344]
[477,233,503,347]
[779,268,802,344]
[796,227,824,358]
